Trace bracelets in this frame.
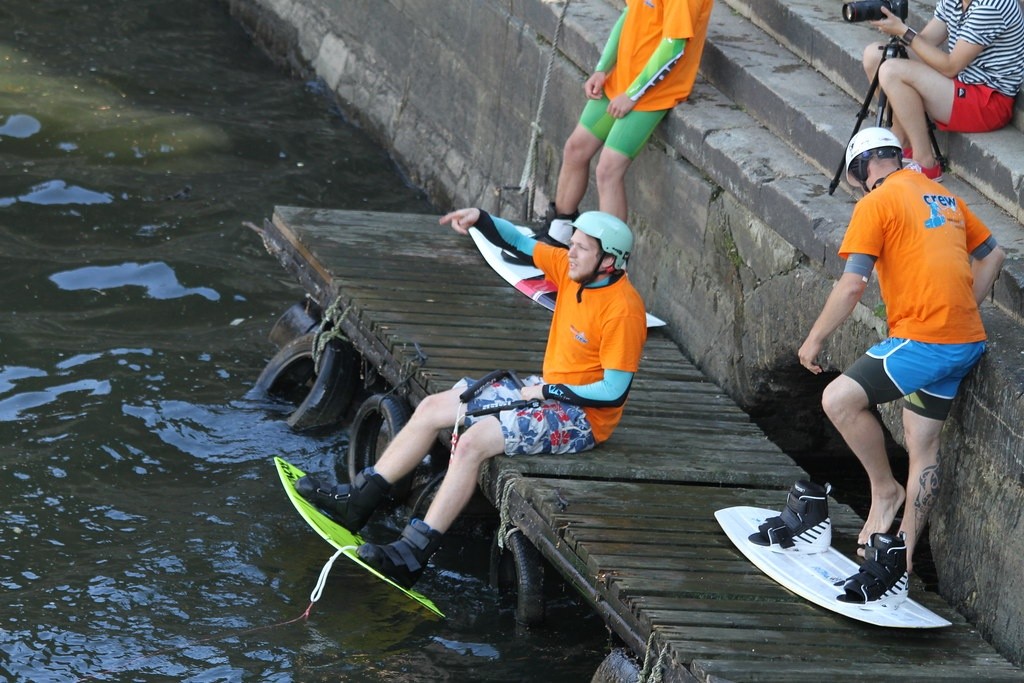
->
[900,28,917,45]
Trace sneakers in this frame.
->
[901,147,913,163]
[904,160,944,183]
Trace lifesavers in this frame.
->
[487,524,547,625]
[256,330,361,431]
[346,391,415,505]
[411,466,485,550]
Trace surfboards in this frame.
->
[467,220,669,329]
[714,480,953,629]
[270,452,449,620]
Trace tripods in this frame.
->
[828,34,949,195]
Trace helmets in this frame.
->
[845,127,902,187]
[563,211,633,270]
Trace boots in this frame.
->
[748,480,832,553]
[835,530,909,611]
[294,466,392,534]
[501,202,580,265]
[356,518,442,590]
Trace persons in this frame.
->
[295,207,646,588]
[503,0,715,266]
[864,0,1024,182]
[798,127,1007,573]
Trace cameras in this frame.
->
[842,0,909,23]
